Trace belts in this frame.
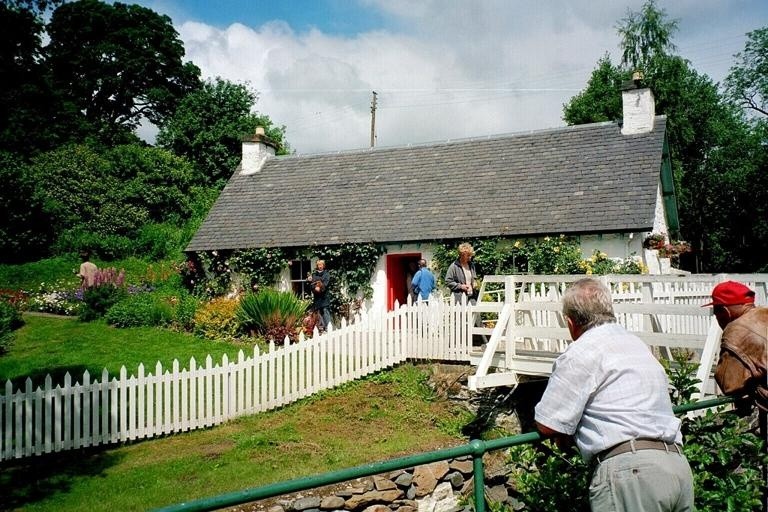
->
[593,439,684,463]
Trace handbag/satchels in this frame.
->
[413,269,423,295]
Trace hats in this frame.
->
[700,280,755,308]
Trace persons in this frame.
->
[412,259,435,304]
[75,252,99,291]
[308,260,330,325]
[533,277,697,511]
[406,262,418,301]
[701,280,767,431]
[444,242,479,303]
